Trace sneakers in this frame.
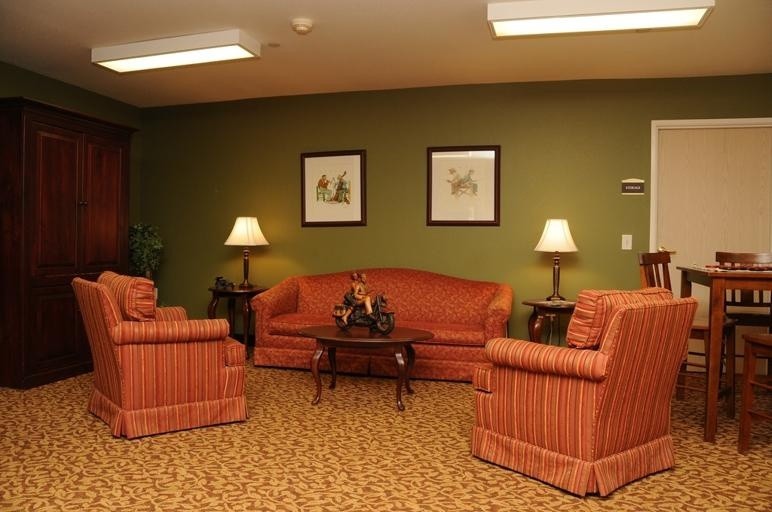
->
[368,314,378,322]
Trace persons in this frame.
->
[447,167,475,199]
[333,271,395,337]
[318,170,353,206]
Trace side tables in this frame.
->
[519,299,577,343]
[204,283,267,361]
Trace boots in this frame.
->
[341,307,353,326]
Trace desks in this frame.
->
[674,264,772,442]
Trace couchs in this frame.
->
[250,267,512,384]
[468,286,694,496]
[69,272,248,439]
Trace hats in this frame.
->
[360,274,367,277]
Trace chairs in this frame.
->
[637,249,737,416]
[739,335,772,452]
[714,252,771,385]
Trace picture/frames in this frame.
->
[426,145,501,227]
[299,149,368,227]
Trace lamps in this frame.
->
[223,216,270,289]
[534,218,578,302]
[485,1,714,38]
[88,28,260,73]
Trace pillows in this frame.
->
[567,285,674,348]
[96,270,157,321]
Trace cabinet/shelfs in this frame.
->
[2,97,139,389]
